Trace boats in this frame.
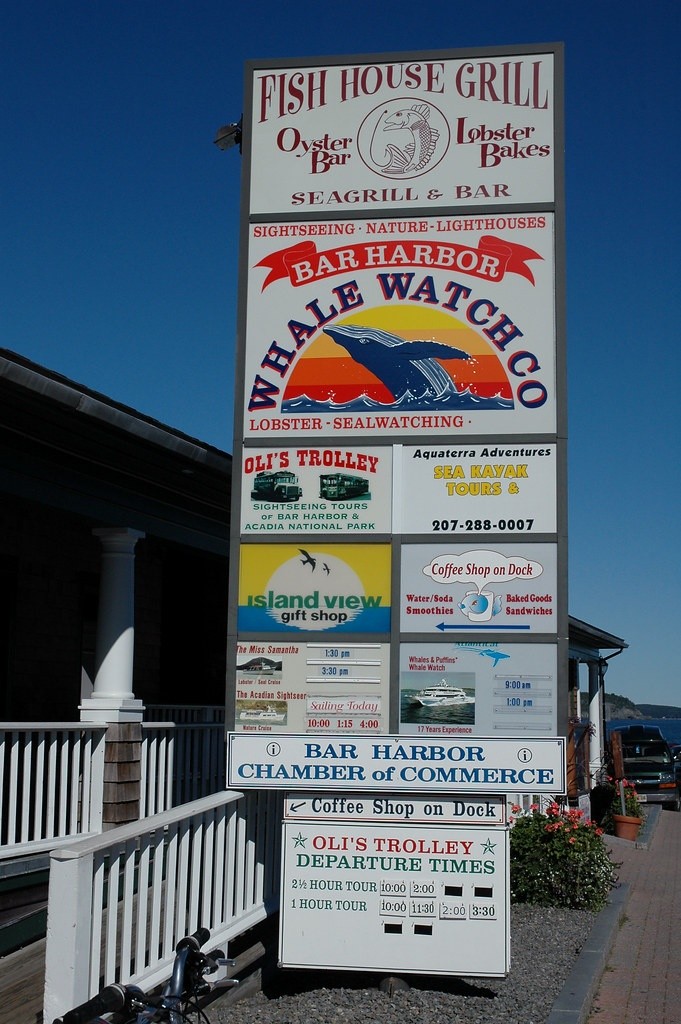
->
[240,705,286,721]
[403,677,475,708]
[242,662,277,675]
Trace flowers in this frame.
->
[604,775,642,817]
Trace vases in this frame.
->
[613,814,641,840]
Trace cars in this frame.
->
[612,725,681,811]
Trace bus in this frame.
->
[253,470,304,501]
[327,474,368,499]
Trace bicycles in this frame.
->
[53,928,240,1024]
